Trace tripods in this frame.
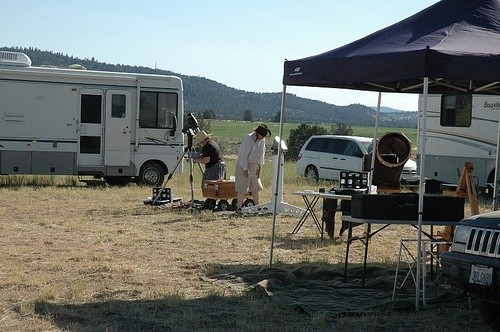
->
[151,132,205,208]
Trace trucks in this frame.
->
[0,51,184,189]
[416,93,500,199]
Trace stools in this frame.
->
[391,239,474,310]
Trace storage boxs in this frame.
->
[203,180,253,199]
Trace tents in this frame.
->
[270,0,500,310]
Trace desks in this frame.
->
[291,191,352,234]
[341,216,459,282]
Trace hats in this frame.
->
[255,123,272,138]
[194,130,212,147]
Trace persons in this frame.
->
[235,123,271,213]
[192,130,227,181]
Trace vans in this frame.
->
[294,133,422,188]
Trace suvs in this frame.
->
[440,208,500,332]
